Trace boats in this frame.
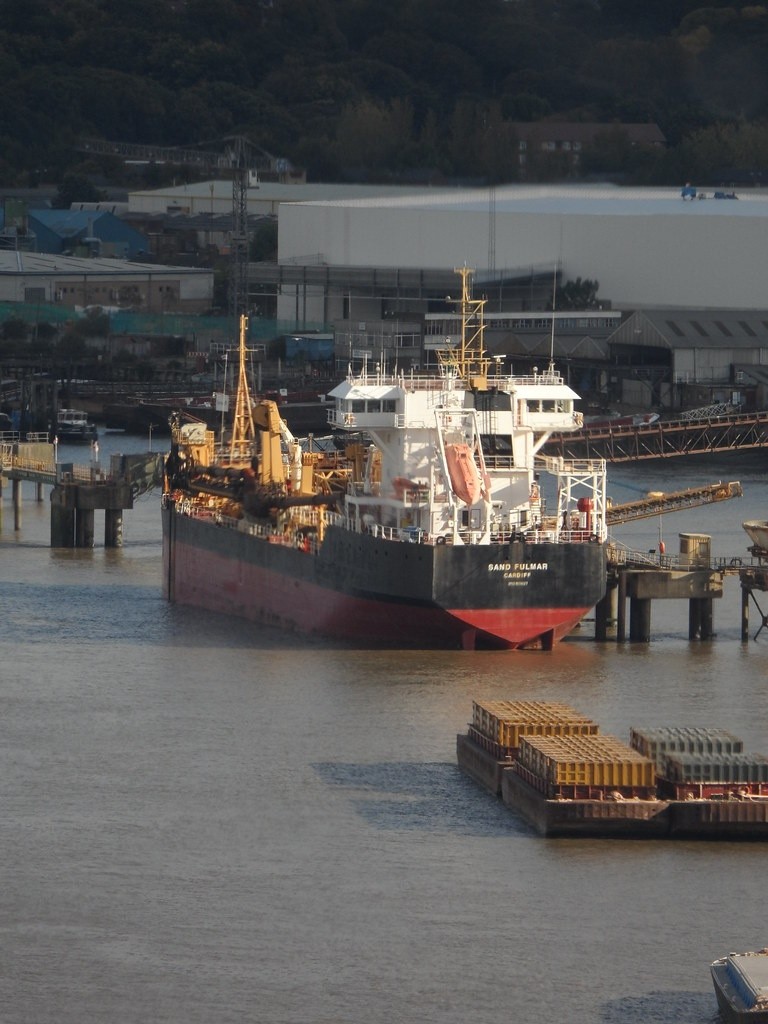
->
[161,267,608,651]
[57,409,98,443]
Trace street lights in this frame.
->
[209,185,214,243]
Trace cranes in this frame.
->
[71,134,290,346]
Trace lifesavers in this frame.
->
[297,533,304,541]
[345,414,354,423]
[266,524,272,530]
[436,536,446,545]
